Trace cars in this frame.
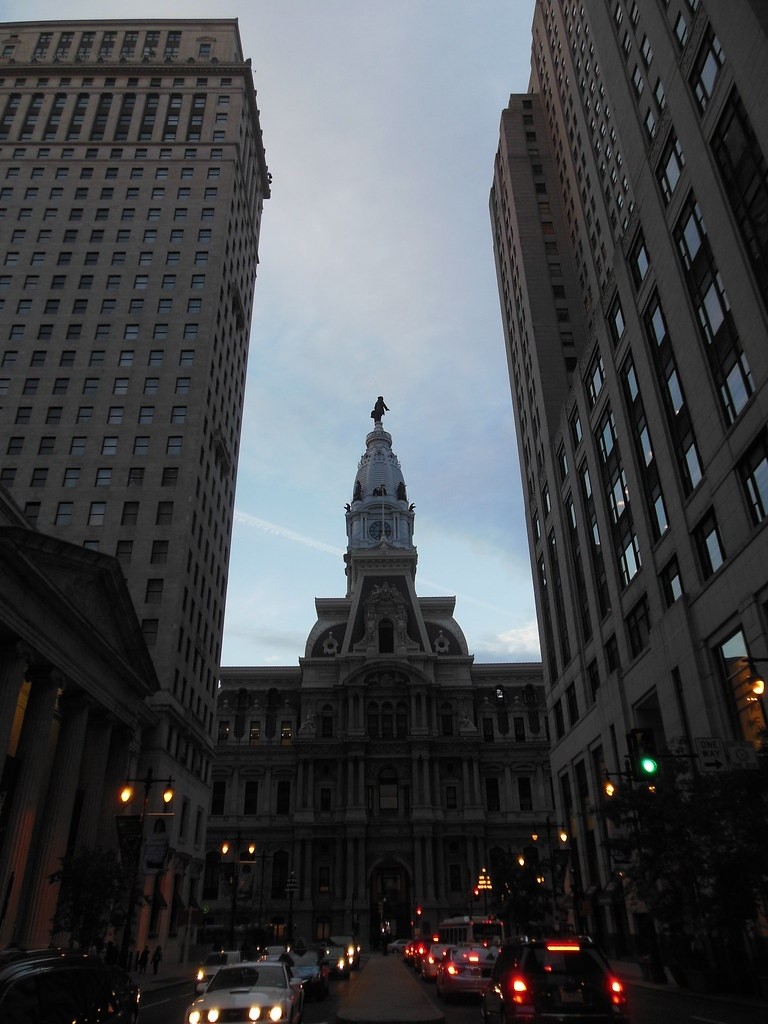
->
[386,935,457,981]
[319,935,361,980]
[184,961,303,1024]
[0,944,141,1023]
[193,945,286,997]
[436,946,502,998]
[483,935,628,1024]
[277,947,330,1001]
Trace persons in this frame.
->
[151,946,162,974]
[371,396,389,422]
[139,945,148,975]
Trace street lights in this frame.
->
[532,816,568,935]
[222,831,255,952]
[478,866,492,916]
[285,870,297,934]
[121,766,177,962]
[495,844,525,935]
[603,759,658,952]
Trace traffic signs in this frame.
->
[697,736,726,773]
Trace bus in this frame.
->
[438,915,506,977]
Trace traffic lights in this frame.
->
[416,905,422,920]
[474,886,480,901]
[629,728,661,780]
